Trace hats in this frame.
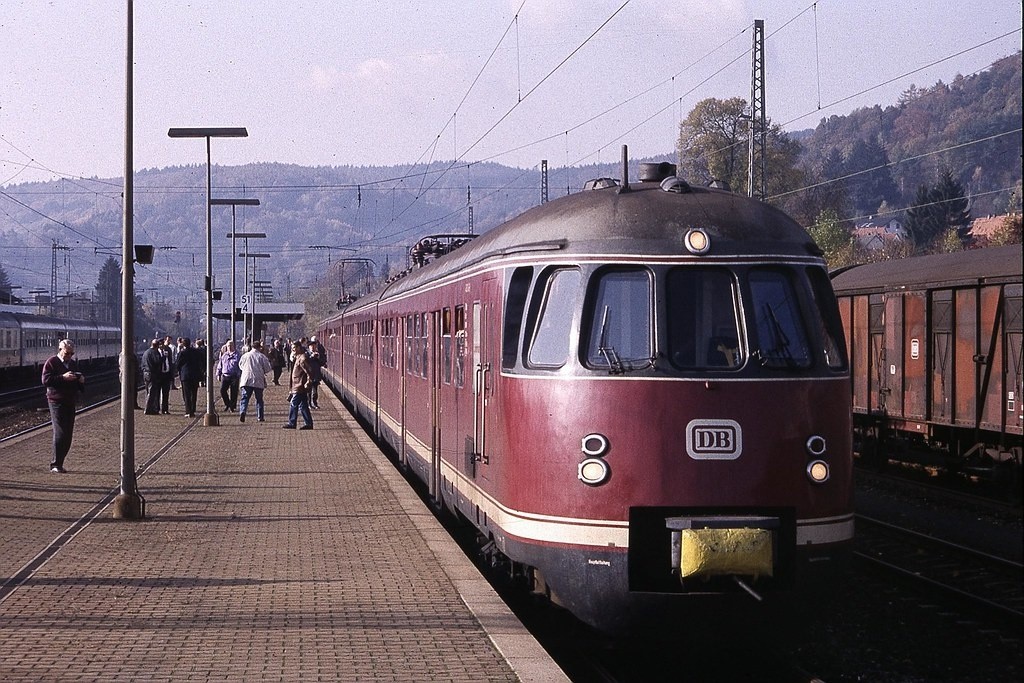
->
[294,339,302,347]
[307,341,315,349]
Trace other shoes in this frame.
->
[224,405,234,413]
[53,467,67,473]
[240,412,246,422]
[300,424,313,430]
[172,386,178,390]
[309,405,319,410]
[258,418,265,422]
[163,411,169,414]
[272,379,280,386]
[283,424,297,430]
[185,413,195,417]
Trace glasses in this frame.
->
[61,348,74,356]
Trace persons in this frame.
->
[216,336,326,413]
[42,339,84,473]
[282,340,314,430]
[238,340,271,423]
[119,336,215,419]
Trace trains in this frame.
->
[823,242,1024,495]
[305,143,862,642]
[0,311,122,405]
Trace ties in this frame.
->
[162,350,167,371]
